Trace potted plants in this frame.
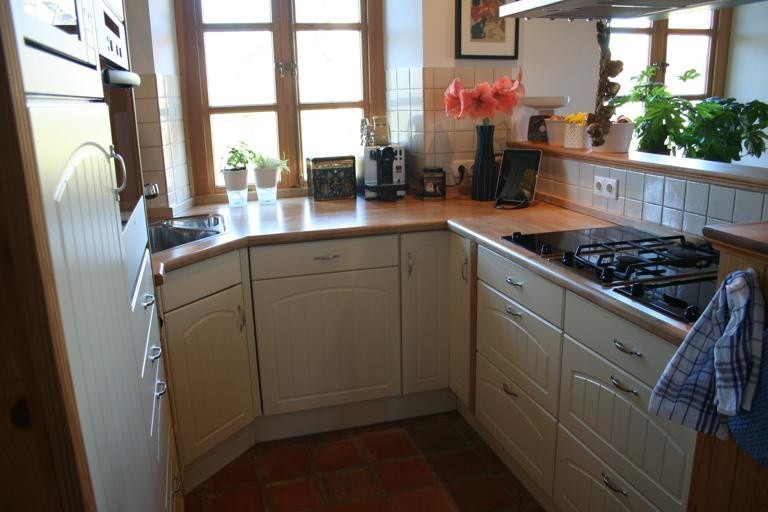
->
[249,148,291,206]
[219,140,253,209]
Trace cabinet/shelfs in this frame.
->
[249,231,400,417]
[552,288,700,512]
[0,97,162,512]
[120,194,186,512]
[474,242,566,499]
[399,229,477,412]
[689,219,766,512]
[156,246,264,470]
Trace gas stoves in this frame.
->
[500,225,720,325]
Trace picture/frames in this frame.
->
[453,0,520,61]
[491,146,543,208]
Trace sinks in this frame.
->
[148,210,225,253]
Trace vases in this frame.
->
[469,124,500,202]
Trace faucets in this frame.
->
[142,180,161,198]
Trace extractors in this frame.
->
[497,0,763,25]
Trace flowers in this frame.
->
[443,64,528,125]
